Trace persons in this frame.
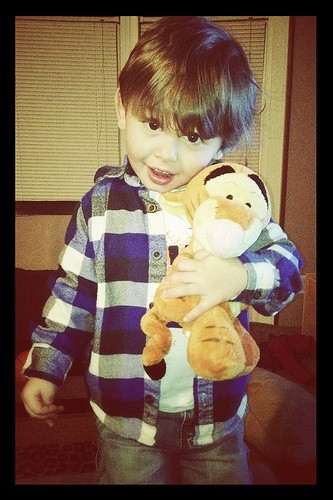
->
[22,15,306,486]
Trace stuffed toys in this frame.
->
[137,162,272,382]
